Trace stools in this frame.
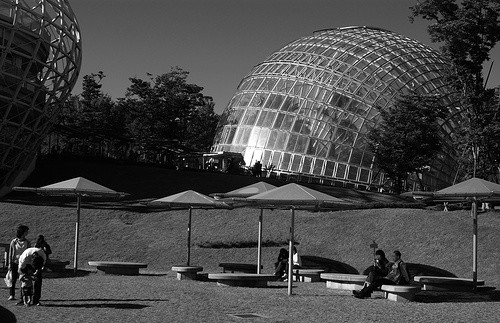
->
[172,266,203,280]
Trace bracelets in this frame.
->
[9,263,12,264]
[42,265,45,266]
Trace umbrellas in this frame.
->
[209,182,279,275]
[245,182,344,298]
[12,177,130,274]
[401,177,500,295]
[140,190,231,266]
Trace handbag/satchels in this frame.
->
[5,269,13,287]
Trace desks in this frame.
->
[0,243,10,266]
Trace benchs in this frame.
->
[283,269,324,282]
[380,284,422,302]
[218,262,264,273]
[432,198,500,212]
[88,261,148,276]
[414,276,485,291]
[44,260,71,272]
[321,273,368,291]
[208,273,276,287]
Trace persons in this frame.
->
[6,225,51,307]
[274,245,302,282]
[353,249,410,299]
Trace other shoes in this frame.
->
[32,300,41,306]
[353,290,364,299]
[15,300,24,305]
[284,279,288,282]
[362,282,370,290]
[282,273,287,278]
[8,295,14,300]
